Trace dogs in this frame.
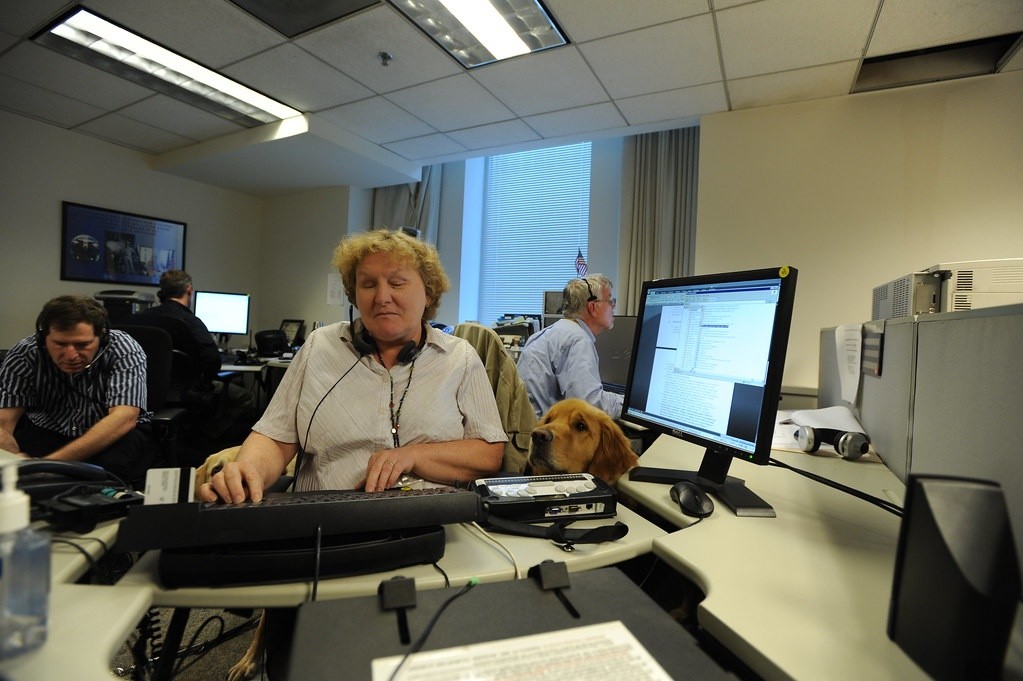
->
[527,399,640,487]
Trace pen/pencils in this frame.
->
[279,360,291,363]
[279,357,293,359]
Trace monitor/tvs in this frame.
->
[194,290,251,352]
[620,266,798,517]
[60,202,187,288]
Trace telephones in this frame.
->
[0,459,126,505]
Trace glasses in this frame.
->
[594,297,617,309]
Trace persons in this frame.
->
[199,224,509,503]
[142,270,255,486]
[0,295,160,485]
[516,272,625,475]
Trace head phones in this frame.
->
[37,309,110,358]
[354,321,427,365]
[794,426,869,459]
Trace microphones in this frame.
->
[74,342,111,378]
[349,304,359,351]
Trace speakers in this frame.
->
[887,474,1023,681]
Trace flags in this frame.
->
[575,249,588,276]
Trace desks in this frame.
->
[0,345,1023,681]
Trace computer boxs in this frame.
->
[593,315,637,388]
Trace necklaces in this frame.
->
[377,348,412,490]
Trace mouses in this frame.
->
[670,481,715,519]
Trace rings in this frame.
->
[386,461,394,467]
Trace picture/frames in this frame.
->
[279,319,305,345]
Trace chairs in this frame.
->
[454,323,539,475]
[110,315,241,455]
